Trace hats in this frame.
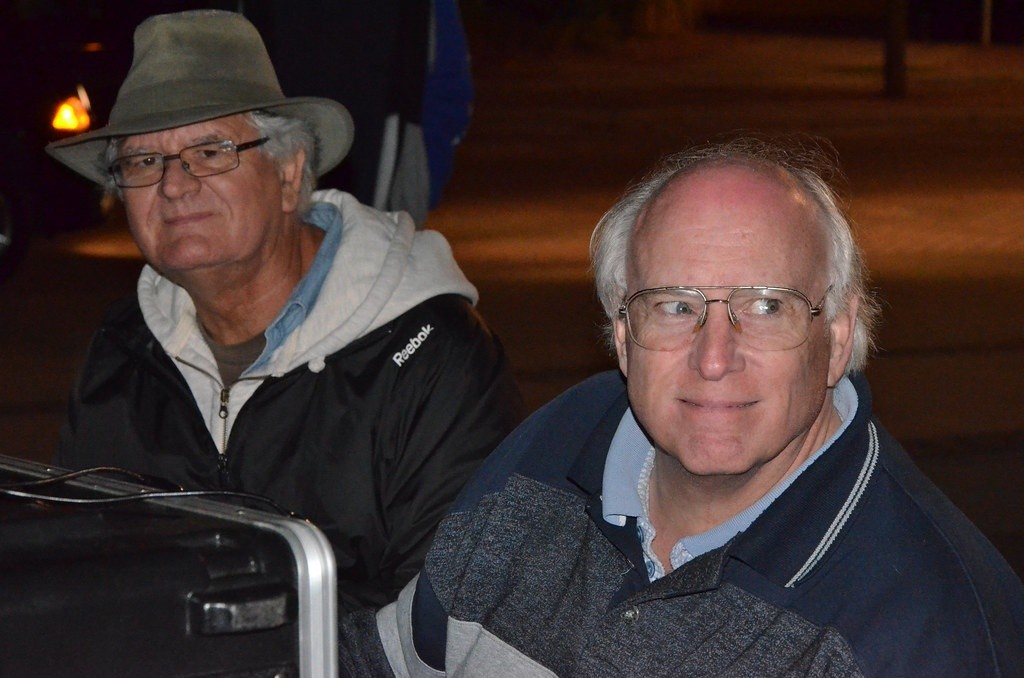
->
[46,10,354,189]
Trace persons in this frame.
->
[334,146,1024,678]
[48,10,520,606]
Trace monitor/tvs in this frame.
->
[0,454,339,678]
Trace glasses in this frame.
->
[618,286,839,354]
[108,136,270,189]
